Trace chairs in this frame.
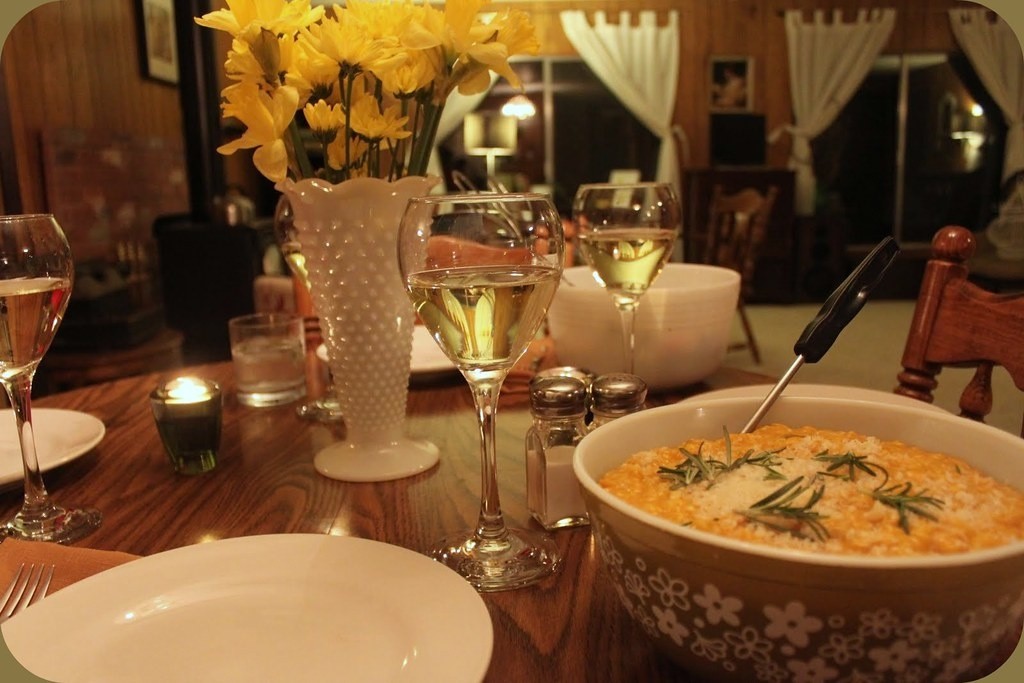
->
[707,184,783,365]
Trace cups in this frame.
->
[228,311,308,409]
[149,379,224,476]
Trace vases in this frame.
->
[269,172,442,481]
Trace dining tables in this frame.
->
[0,356,803,683]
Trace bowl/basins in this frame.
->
[548,263,742,393]
[573,395,1024,683]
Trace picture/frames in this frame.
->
[703,53,754,114]
[133,0,180,88]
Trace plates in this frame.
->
[316,324,461,376]
[0,532,495,683]
[0,407,106,486]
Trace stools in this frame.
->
[41,323,183,394]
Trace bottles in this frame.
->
[524,365,591,532]
[588,374,648,431]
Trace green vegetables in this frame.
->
[656,425,946,543]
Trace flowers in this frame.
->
[193,0,541,185]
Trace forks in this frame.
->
[0,563,55,623]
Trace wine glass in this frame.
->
[396,192,565,594]
[572,182,683,373]
[273,195,347,424]
[0,213,104,545]
